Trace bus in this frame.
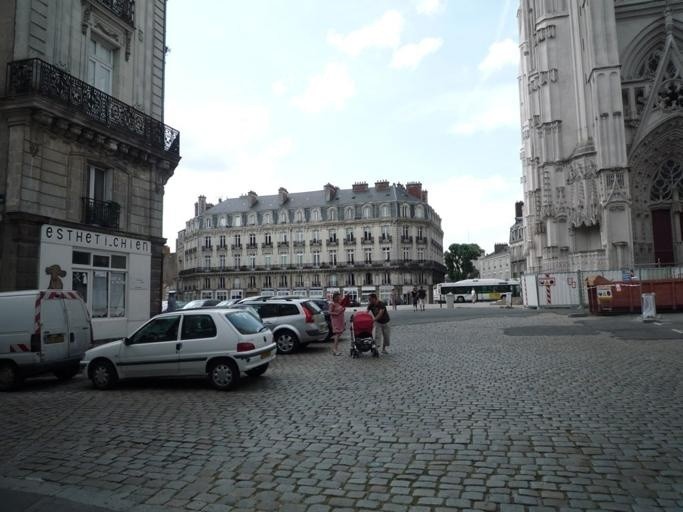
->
[433,279,520,302]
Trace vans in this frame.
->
[1,289,95,391]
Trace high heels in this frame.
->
[333,352,344,356]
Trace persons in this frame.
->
[327,291,347,356]
[366,293,391,354]
[410,285,426,312]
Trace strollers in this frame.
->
[350,311,380,359]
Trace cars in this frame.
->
[80,295,346,391]
